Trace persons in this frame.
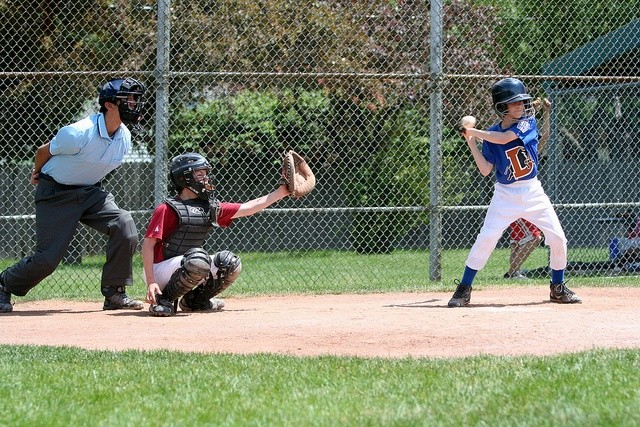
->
[142,150,316,316]
[504,97,553,279]
[0,80,144,312]
[447,77,582,306]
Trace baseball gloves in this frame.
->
[282,150,315,199]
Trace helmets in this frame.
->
[491,78,535,124]
[99,77,146,125]
[169,152,216,201]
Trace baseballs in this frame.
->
[463,115,476,130]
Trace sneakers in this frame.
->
[549,278,582,303]
[0,284,13,312]
[148,297,174,316]
[179,296,225,313]
[102,286,144,310]
[504,272,532,280]
[447,279,473,308]
